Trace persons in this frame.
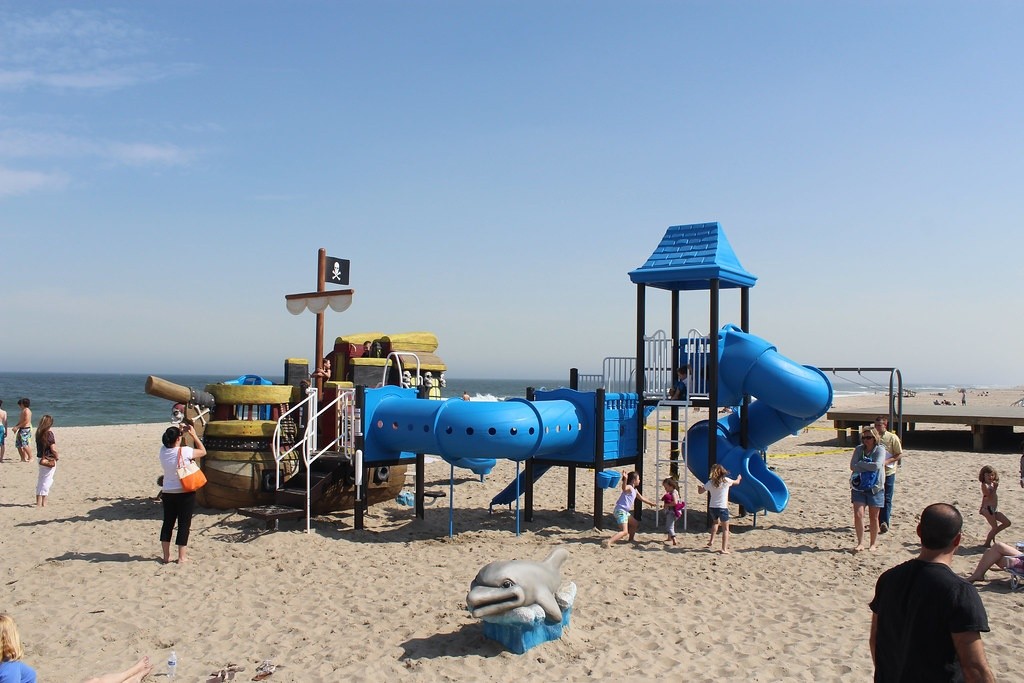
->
[311,357,332,394]
[965,543,1024,586]
[11,397,35,463]
[603,468,658,548]
[849,424,887,552]
[697,464,742,554]
[33,414,60,507]
[1018,453,1024,488]
[0,612,154,683]
[669,364,694,401]
[462,391,471,401]
[158,422,207,563]
[361,340,372,358]
[659,476,680,546]
[979,464,1011,547]
[962,391,967,406]
[872,417,902,532]
[933,400,952,406]
[0,400,8,463]
[867,505,999,682]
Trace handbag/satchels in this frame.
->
[177,446,214,490]
[39,453,56,468]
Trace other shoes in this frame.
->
[880,522,888,533]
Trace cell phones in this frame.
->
[183,426,190,432]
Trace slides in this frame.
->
[681,328,834,514]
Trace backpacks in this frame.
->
[848,444,880,493]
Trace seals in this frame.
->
[465,548,569,622]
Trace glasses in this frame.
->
[860,435,874,440]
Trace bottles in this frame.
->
[167,652,177,678]
